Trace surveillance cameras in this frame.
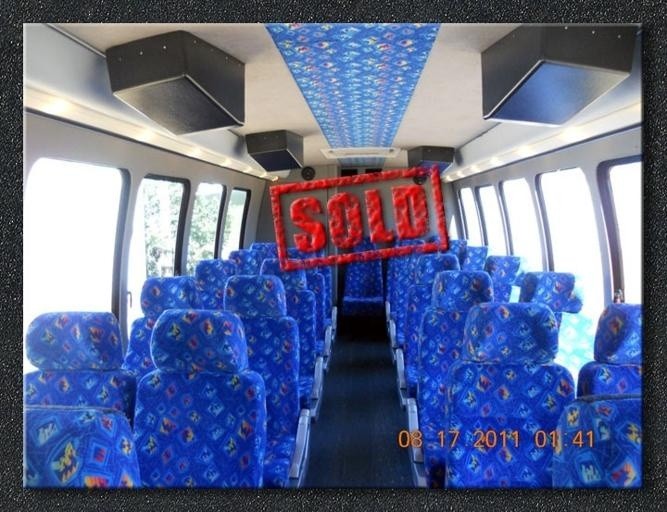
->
[302,166,315,180]
[413,176,427,185]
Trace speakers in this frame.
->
[106,30,246,138]
[481,23,637,126]
[409,146,454,176]
[246,129,304,172]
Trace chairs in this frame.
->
[24,239,642,488]
